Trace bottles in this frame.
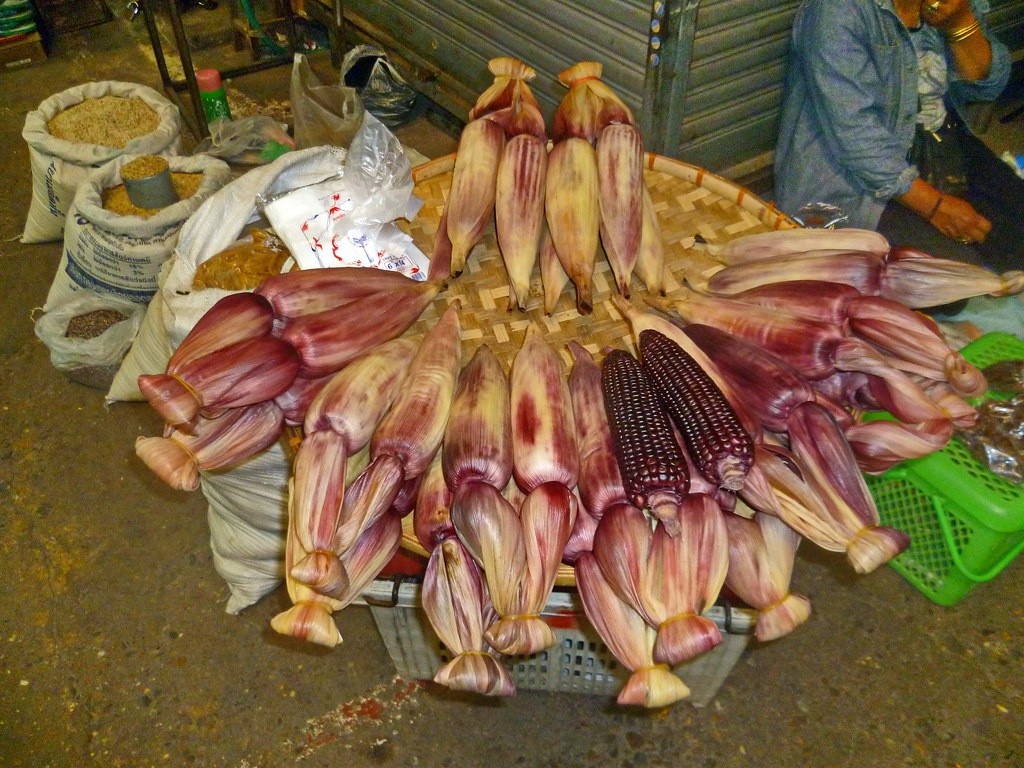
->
[195,69,232,124]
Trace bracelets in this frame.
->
[924,192,945,224]
[944,20,981,44]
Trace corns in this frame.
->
[255,118,894,562]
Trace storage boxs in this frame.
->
[860,330,1024,607]
[362,578,760,707]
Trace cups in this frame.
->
[122,166,179,209]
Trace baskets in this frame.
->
[359,573,759,708]
[862,331,1024,607]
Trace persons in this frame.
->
[775,0,1024,275]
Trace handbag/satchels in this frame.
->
[338,45,419,131]
[192,116,297,164]
[289,52,366,151]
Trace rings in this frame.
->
[959,238,969,245]
[926,0,941,14]
